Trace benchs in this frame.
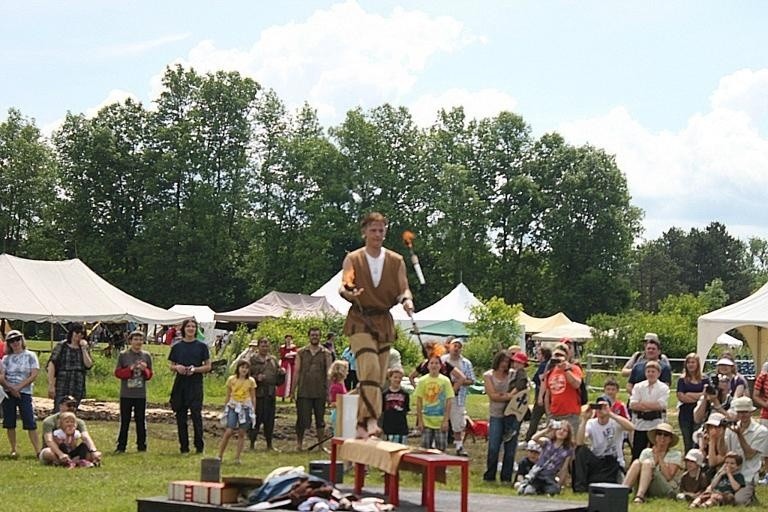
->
[330,436,470,512]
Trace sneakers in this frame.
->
[114,449,125,454]
[514,481,539,495]
[502,428,518,442]
[66,458,95,469]
[454,446,468,457]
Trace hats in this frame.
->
[647,423,679,448]
[730,395,758,413]
[56,395,79,407]
[640,332,659,343]
[508,345,528,364]
[248,339,258,346]
[560,338,573,343]
[522,439,542,454]
[5,330,25,341]
[551,343,570,360]
[685,448,706,467]
[705,412,725,430]
[443,335,464,349]
[596,397,612,406]
[714,358,735,366]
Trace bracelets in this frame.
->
[564,367,573,373]
[401,297,413,304]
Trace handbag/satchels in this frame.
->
[580,379,588,404]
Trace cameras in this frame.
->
[722,418,739,425]
[551,358,562,365]
[708,374,719,391]
[590,403,604,410]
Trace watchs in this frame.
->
[89,448,97,452]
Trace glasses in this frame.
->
[656,432,669,437]
[66,404,77,407]
[8,337,20,343]
[75,330,86,335]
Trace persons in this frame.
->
[215,359,257,465]
[622,423,683,506]
[294,327,476,458]
[676,350,767,508]
[38,395,101,468]
[0,330,41,459]
[626,340,672,420]
[677,353,710,455]
[339,211,415,438]
[511,341,584,497]
[167,319,212,457]
[629,361,670,461]
[593,379,631,421]
[115,330,153,455]
[44,323,94,413]
[483,344,532,482]
[621,332,670,377]
[276,334,297,403]
[572,395,634,494]
[245,336,281,453]
[52,412,95,470]
